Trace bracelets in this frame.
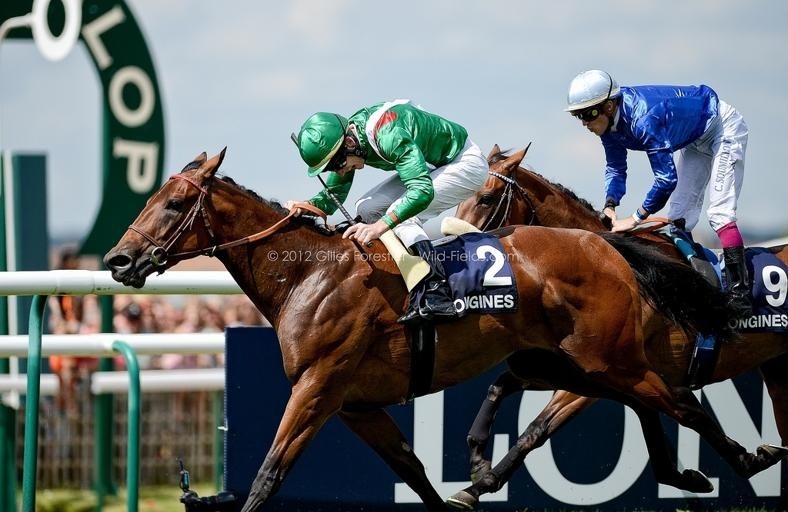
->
[632,208,647,224]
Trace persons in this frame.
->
[279,97,491,328]
[562,68,754,319]
[40,250,269,489]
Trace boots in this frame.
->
[711,246,753,311]
[396,240,456,323]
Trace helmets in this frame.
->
[297,112,349,177]
[563,70,621,113]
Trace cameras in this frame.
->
[126,305,141,319]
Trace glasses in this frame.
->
[321,114,347,172]
[571,72,612,121]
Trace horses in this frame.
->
[453,143,788,510]
[101,145,788,511]
